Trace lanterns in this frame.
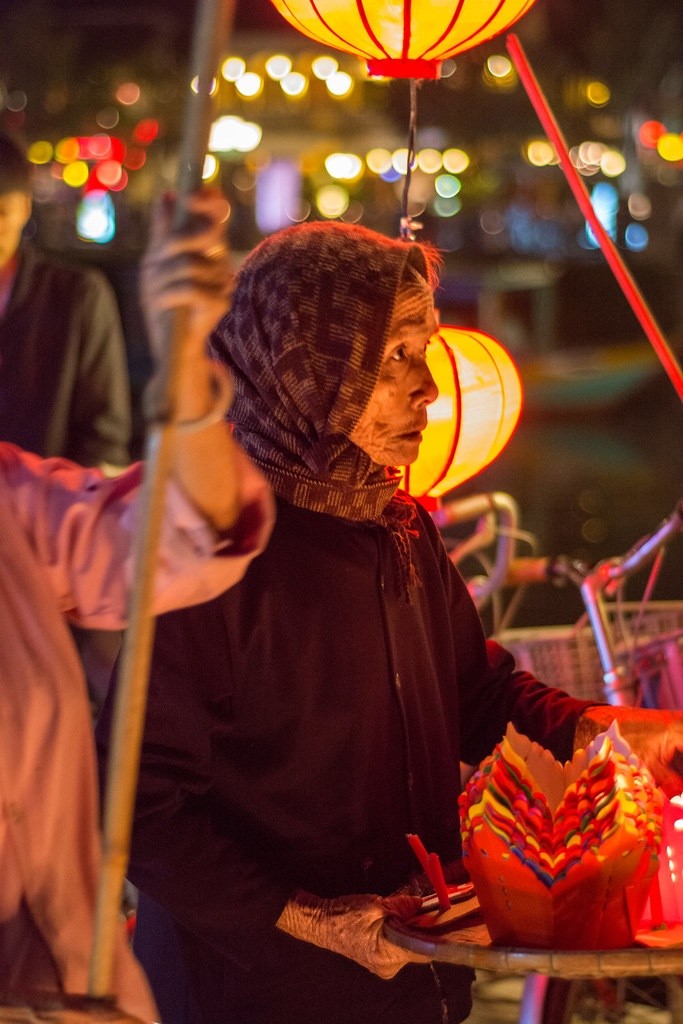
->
[396,329,526,516]
[268,0,537,82]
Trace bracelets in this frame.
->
[143,361,237,435]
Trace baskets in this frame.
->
[483,609,676,703]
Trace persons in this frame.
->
[0,132,280,1024]
[94,220,683,1024]
